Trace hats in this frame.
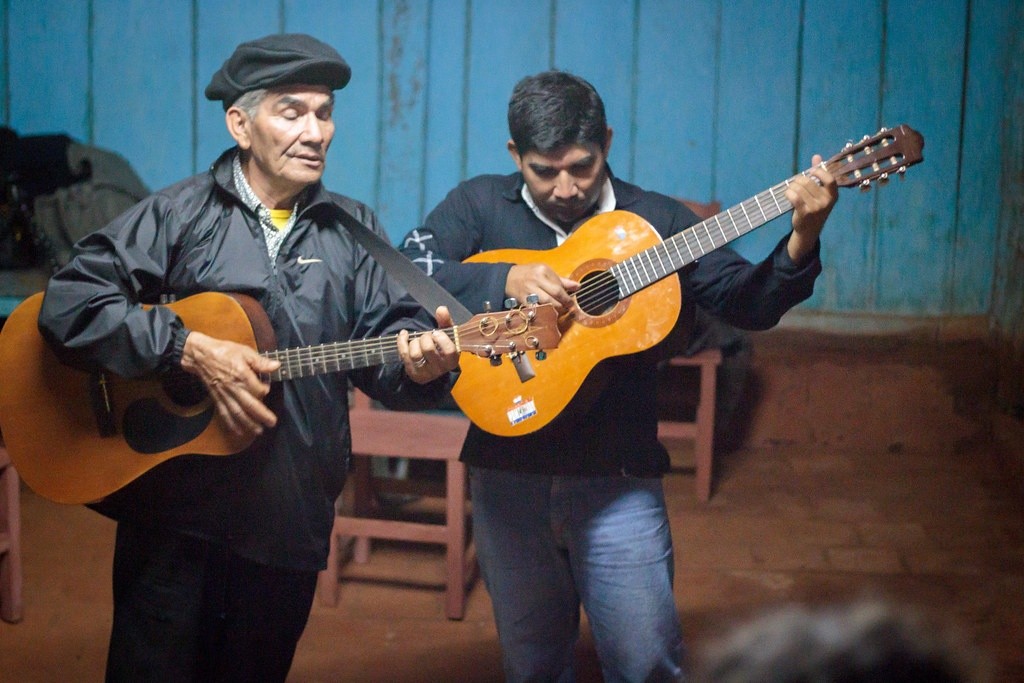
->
[205,33,351,112]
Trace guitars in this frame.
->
[0,277,563,507]
[428,120,926,439]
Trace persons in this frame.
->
[396,70,840,683]
[36,31,461,683]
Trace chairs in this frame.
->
[657,199,722,502]
[316,385,480,620]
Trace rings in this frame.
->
[412,356,427,369]
